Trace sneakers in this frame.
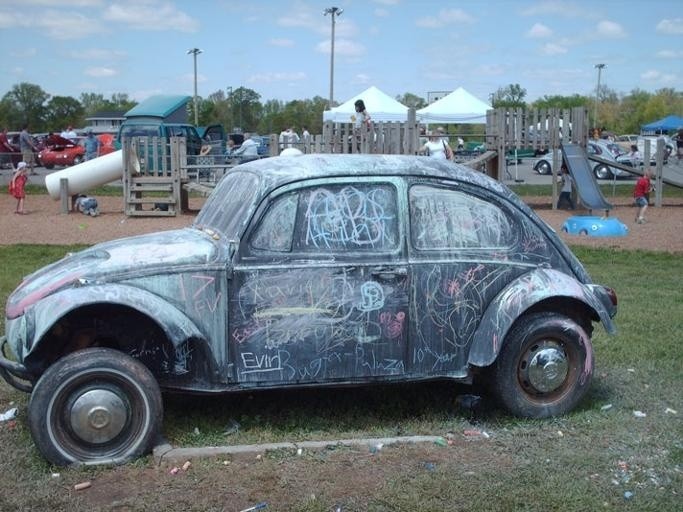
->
[13,209,27,216]
[634,217,649,224]
[89,208,100,217]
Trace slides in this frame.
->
[559,143,614,210]
[45,147,140,201]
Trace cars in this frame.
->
[454,128,683,179]
[1,155,620,470]
[2,94,281,181]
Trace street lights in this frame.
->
[319,5,343,106]
[184,45,204,128]
[593,62,607,129]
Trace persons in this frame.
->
[0,126,14,175]
[73,194,100,217]
[83,128,100,162]
[61,125,77,139]
[628,144,640,157]
[196,145,212,181]
[19,123,39,176]
[233,133,257,164]
[351,99,371,131]
[418,126,464,162]
[279,124,311,154]
[557,164,576,209]
[7,161,28,214]
[634,168,655,224]
[224,139,235,164]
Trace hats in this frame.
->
[16,162,28,170]
[200,144,211,157]
